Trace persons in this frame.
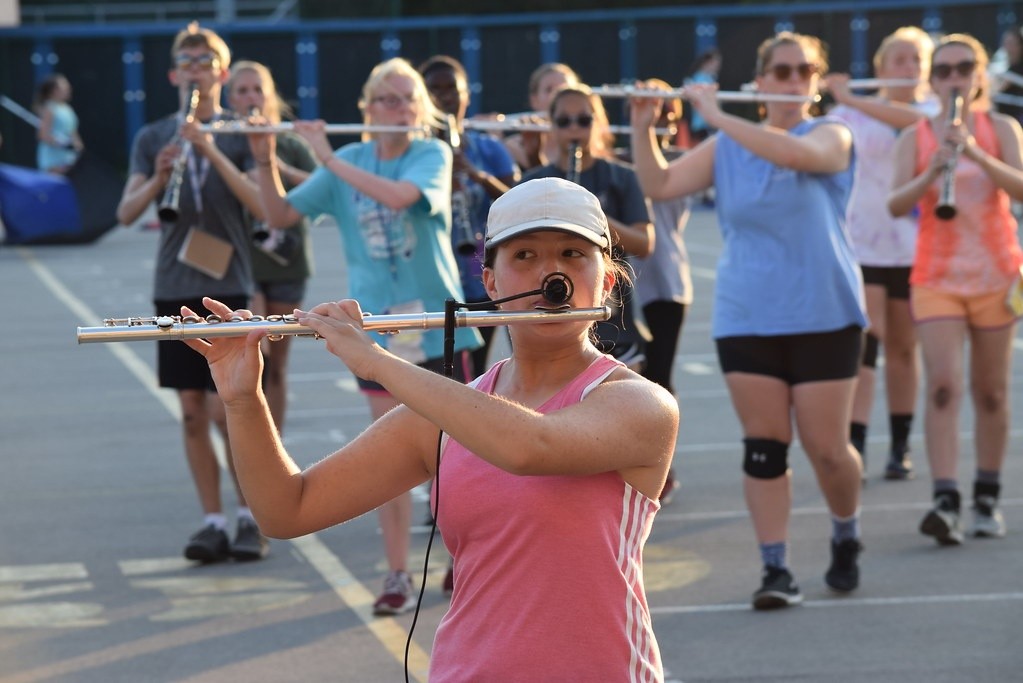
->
[418,57,693,506]
[119,31,321,561]
[32,74,84,172]
[629,25,1023,608]
[248,56,485,616]
[177,177,679,683]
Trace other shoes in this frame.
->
[656,471,678,503]
[885,453,912,480]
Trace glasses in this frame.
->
[370,92,416,108]
[549,112,593,129]
[176,54,225,70]
[933,59,978,79]
[763,63,820,81]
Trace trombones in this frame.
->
[563,135,587,188]
[436,107,482,256]
[930,83,966,223]
[153,77,202,221]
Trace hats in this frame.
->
[482,177,611,269]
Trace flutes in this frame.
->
[72,308,614,348]
[201,69,1021,153]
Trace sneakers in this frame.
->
[920,507,963,546]
[443,557,456,598]
[375,570,417,614]
[753,563,803,609]
[825,537,860,594]
[184,526,231,564]
[972,495,1006,539]
[230,517,270,559]
